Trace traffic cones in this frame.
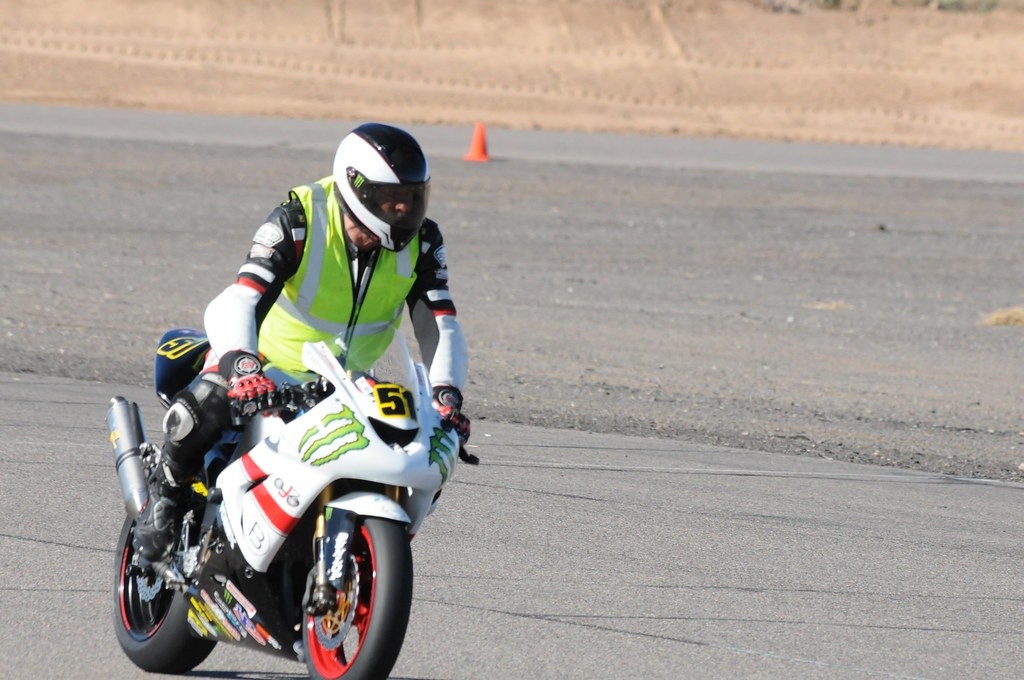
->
[463,123,488,162]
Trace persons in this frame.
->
[131,123,467,564]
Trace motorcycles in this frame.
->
[105,324,481,680]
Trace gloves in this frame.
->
[225,373,276,414]
[433,387,467,439]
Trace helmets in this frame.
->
[334,122,431,251]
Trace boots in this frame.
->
[133,443,200,560]
